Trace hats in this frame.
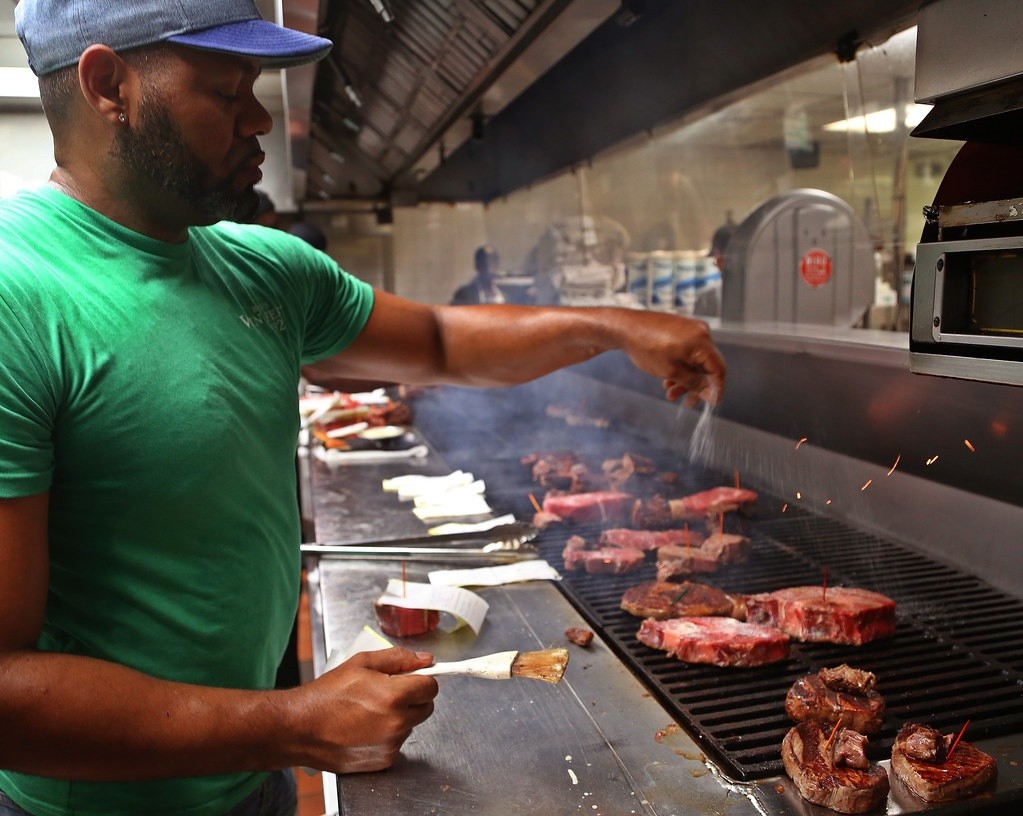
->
[14,0,333,75]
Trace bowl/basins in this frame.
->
[374,599,440,637]
[356,424,406,449]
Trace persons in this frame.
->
[1,0,730,816]
[450,246,513,305]
[707,209,742,258]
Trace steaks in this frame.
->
[513,449,998,813]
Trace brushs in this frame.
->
[402,646,569,686]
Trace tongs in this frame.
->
[300,520,539,567]
